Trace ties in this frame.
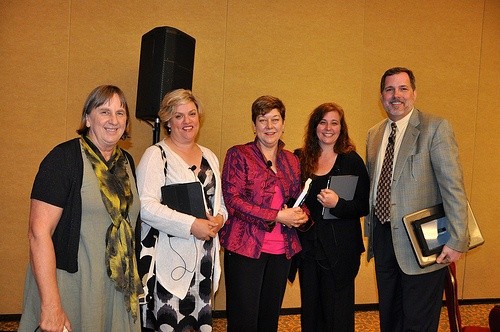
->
[375,123,397,224]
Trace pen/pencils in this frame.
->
[322,179,330,215]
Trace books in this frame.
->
[161,181,208,238]
[321,175,358,220]
[283,177,313,228]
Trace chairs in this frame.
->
[444,261,491,332]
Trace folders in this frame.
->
[322,175,359,219]
[161,182,210,238]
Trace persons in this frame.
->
[364,66,469,332]
[135,88,228,332]
[16,85,144,332]
[288,104,369,332]
[220,95,314,332]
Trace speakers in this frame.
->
[136,26,195,121]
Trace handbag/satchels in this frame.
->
[137,145,167,287]
